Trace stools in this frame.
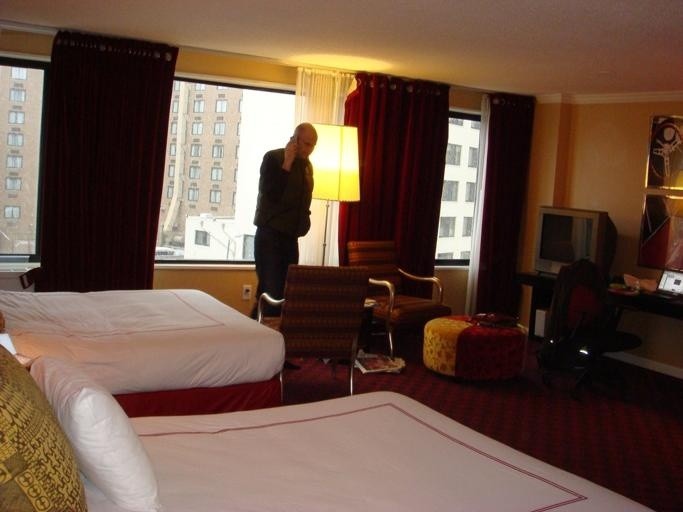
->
[422,313,530,387]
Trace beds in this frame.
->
[25,374,664,512]
[0,287,288,415]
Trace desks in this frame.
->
[515,271,592,344]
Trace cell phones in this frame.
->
[290,136,298,144]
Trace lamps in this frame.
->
[306,120,361,266]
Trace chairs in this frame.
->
[255,240,454,406]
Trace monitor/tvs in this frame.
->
[534,206,617,277]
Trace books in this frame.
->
[356,356,394,376]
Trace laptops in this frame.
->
[647,269,683,303]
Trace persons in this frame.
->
[242,122,318,368]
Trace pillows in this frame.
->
[0,337,162,512]
